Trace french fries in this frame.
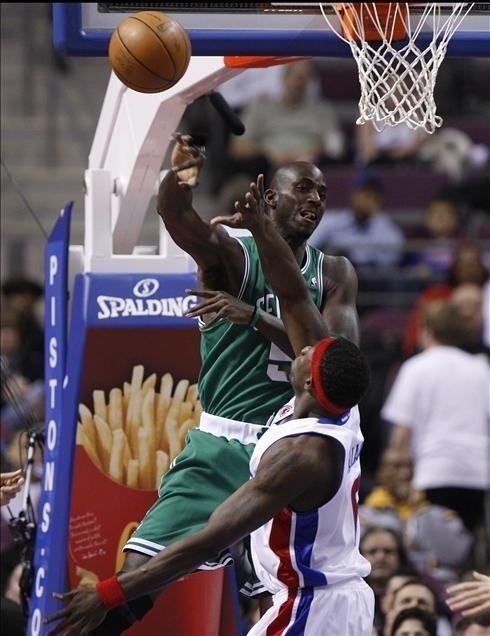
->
[78,364,203,491]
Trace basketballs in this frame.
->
[109,10,190,92]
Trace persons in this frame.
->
[99,133,361,636]
[308,105,490,636]
[228,62,342,169]
[0,276,47,636]
[43,172,376,635]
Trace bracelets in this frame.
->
[248,305,261,328]
[96,576,127,611]
